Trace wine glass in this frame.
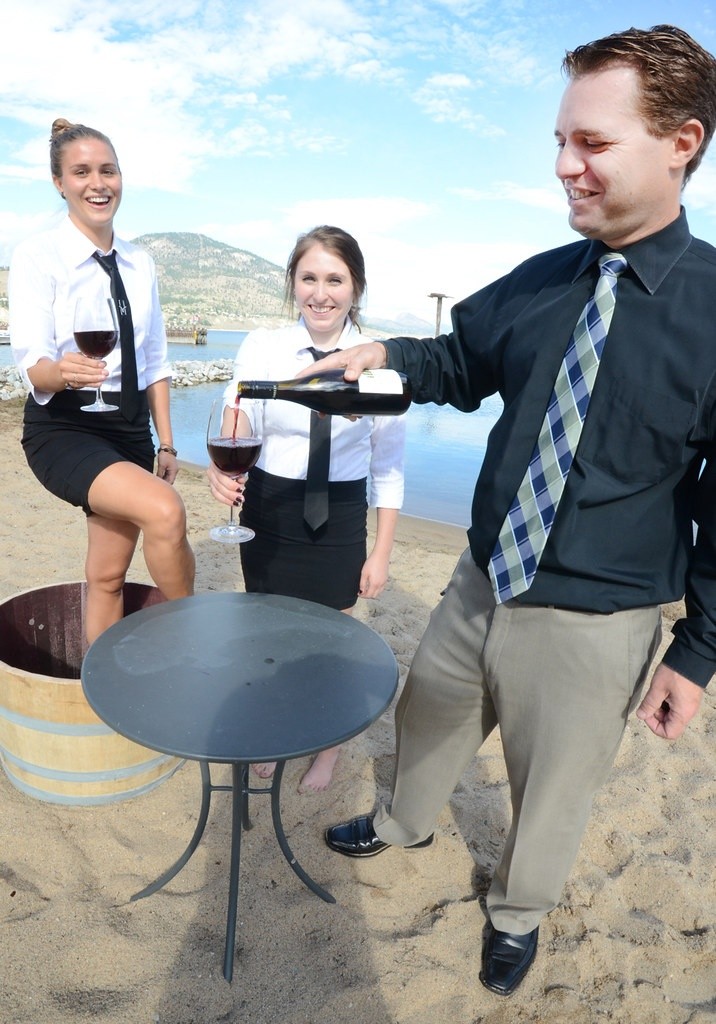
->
[73,296,120,412]
[205,397,263,544]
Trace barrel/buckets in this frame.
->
[0,579,187,806]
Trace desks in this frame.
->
[80,591,398,982]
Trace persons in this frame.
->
[9,118,195,646]
[207,226,405,793]
[293,24,716,996]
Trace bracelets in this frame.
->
[157,443,178,457]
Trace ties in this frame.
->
[489,253,628,604]
[303,347,341,531]
[93,250,140,422]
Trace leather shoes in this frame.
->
[324,814,434,857]
[479,925,540,996]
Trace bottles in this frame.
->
[237,369,414,415]
